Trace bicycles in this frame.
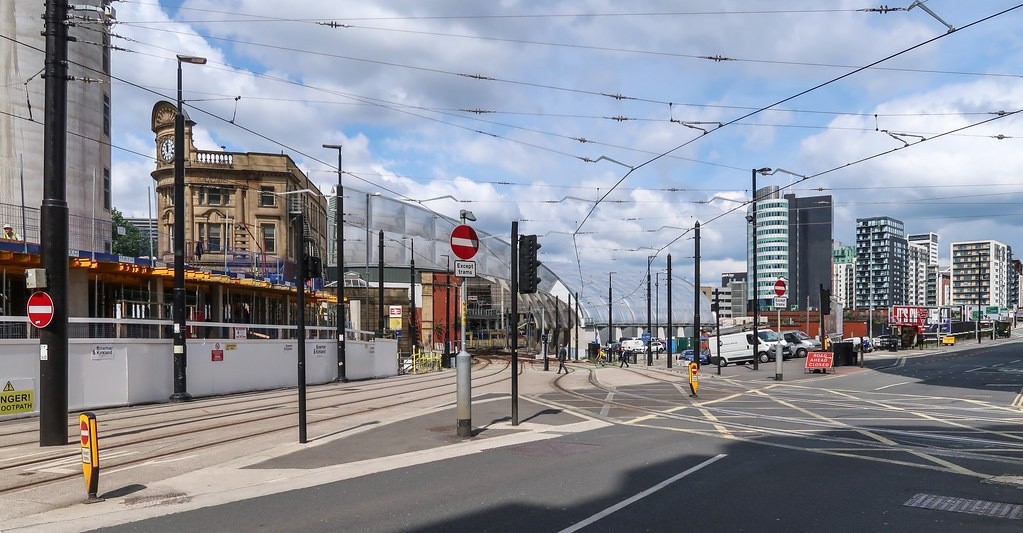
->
[594,357,605,368]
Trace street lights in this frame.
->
[751,168,773,371]
[321,143,352,383]
[647,255,657,366]
[608,272,618,362]
[168,53,207,401]
[978,253,990,343]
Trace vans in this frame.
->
[709,329,790,368]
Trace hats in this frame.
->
[560,343,563,346]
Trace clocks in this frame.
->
[161,135,175,164]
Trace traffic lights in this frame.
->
[519,234,542,294]
[823,289,832,314]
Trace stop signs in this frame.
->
[27,291,54,328]
[774,281,787,295]
[450,225,480,258]
[80,421,89,444]
[692,365,697,375]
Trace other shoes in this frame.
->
[627,365,629,368]
[565,372,568,375]
[620,366,623,368]
[557,372,560,374]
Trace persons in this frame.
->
[557,343,569,374]
[2,224,23,241]
[917,331,924,350]
[596,348,629,367]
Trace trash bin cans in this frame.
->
[625,352,637,364]
[833,342,853,367]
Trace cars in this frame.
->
[679,350,709,365]
[600,334,666,354]
[780,330,822,358]
